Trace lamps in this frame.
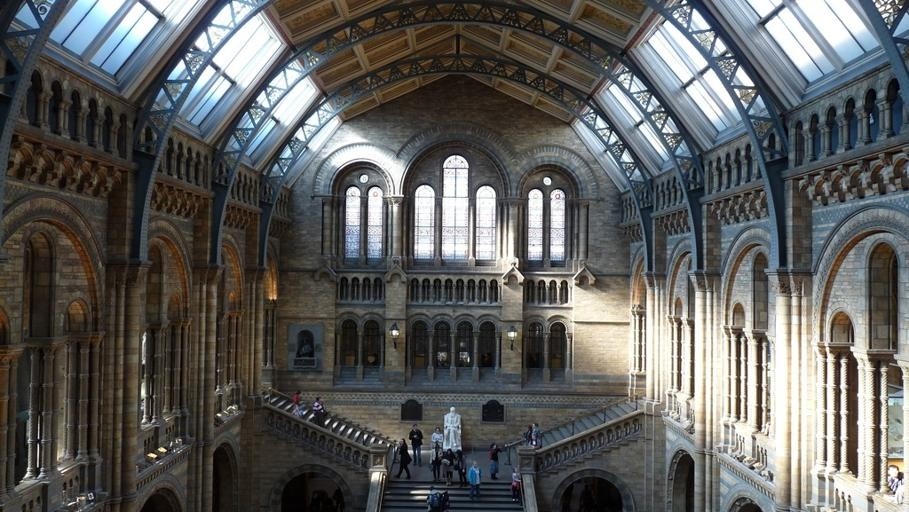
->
[507,326,518,352]
[389,323,400,350]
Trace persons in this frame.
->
[887,464,904,504]
[443,406,461,451]
[393,417,549,512]
[291,390,304,418]
[313,395,326,423]
